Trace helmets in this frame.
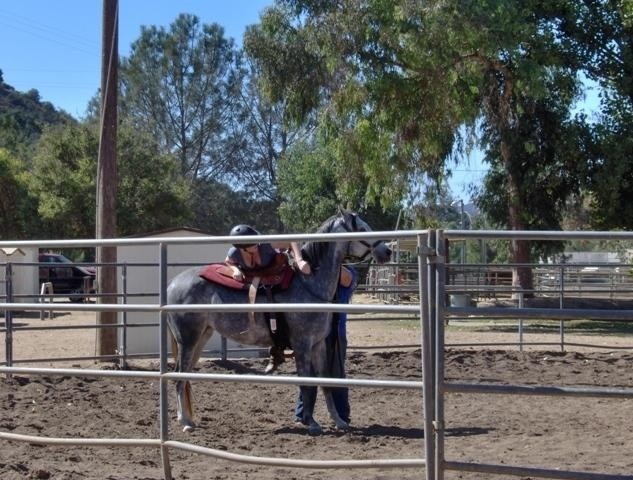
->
[230,225,259,248]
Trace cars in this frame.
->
[38,253,95,302]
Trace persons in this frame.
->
[224,222,312,375]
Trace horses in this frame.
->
[167,201,392,436]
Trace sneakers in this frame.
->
[264,350,285,374]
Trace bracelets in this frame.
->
[294,257,304,263]
